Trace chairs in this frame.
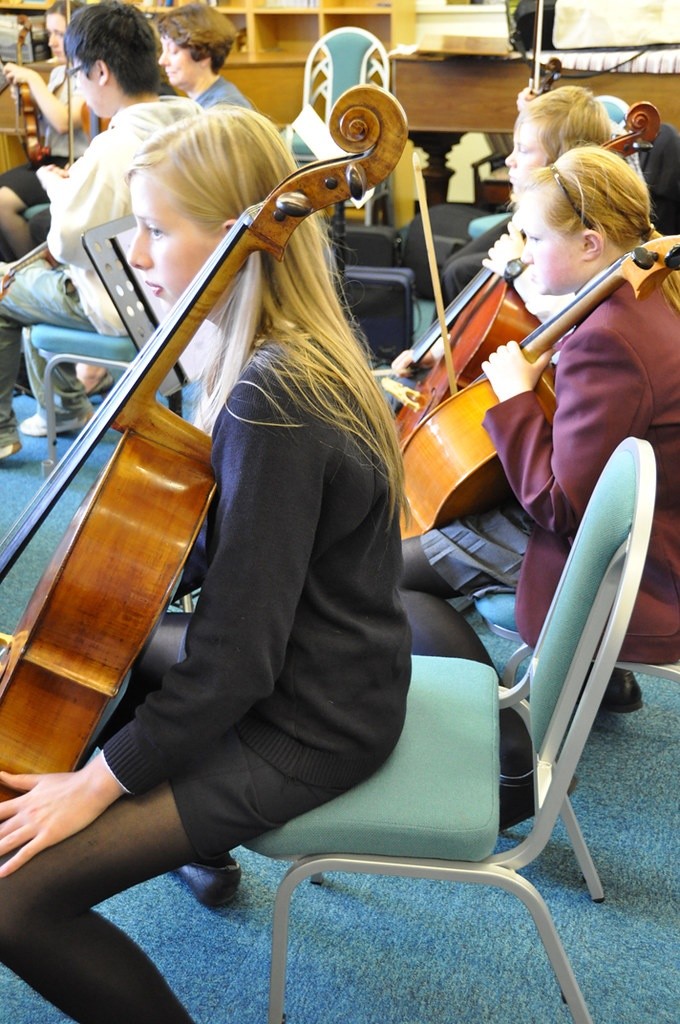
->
[30,323,139,459]
[466,94,632,241]
[242,437,657,1024]
[281,26,390,227]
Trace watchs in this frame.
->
[504,257,529,283]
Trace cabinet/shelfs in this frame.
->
[0,0,415,128]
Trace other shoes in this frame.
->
[576,669,643,714]
[0,441,22,459]
[172,862,241,906]
[498,768,578,832]
[20,410,94,436]
[87,371,114,398]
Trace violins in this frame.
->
[80,101,113,145]
[13,14,53,161]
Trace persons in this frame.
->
[1,0,255,460]
[0,108,414,1024]
[374,86,680,690]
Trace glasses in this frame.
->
[66,61,95,79]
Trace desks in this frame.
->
[389,52,680,135]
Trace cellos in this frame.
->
[397,232,680,540]
[1,86,408,806]
[392,99,661,461]
